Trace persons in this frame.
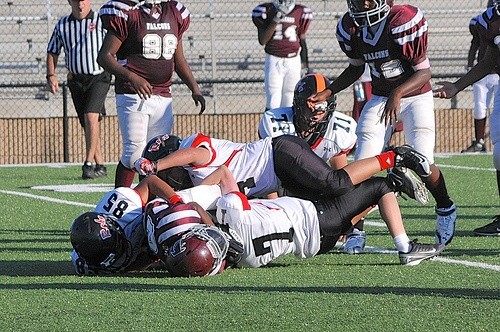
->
[70,134,457,277]
[432,0,500,236]
[258,74,358,169]
[46,0,117,179]
[252,0,312,112]
[97,0,206,188]
[307,0,456,253]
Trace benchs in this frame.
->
[0,0,500,119]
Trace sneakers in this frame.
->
[386,143,430,174]
[434,199,457,246]
[399,238,446,266]
[473,215,500,236]
[343,227,367,254]
[460,141,487,153]
[81,161,108,180]
[388,168,429,205]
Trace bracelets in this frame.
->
[46,74,54,78]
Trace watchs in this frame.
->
[275,11,282,19]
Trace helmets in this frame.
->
[293,73,337,134]
[141,136,183,176]
[70,211,134,276]
[347,0,394,28]
[272,0,295,15]
[165,224,232,276]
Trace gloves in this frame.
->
[134,158,156,175]
[137,250,168,278]
[224,234,245,269]
[273,5,289,23]
